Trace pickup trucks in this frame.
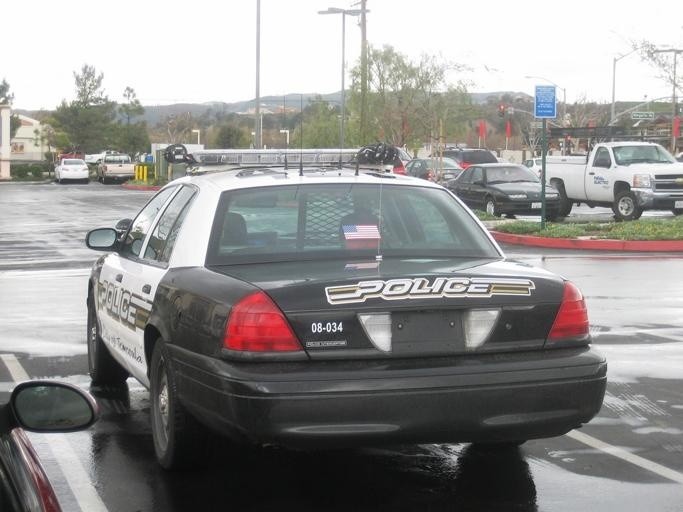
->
[523,141,683,222]
[96,151,135,183]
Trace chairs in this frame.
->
[219,212,249,246]
[338,213,379,240]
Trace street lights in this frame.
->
[610,43,656,127]
[525,75,566,122]
[650,49,683,156]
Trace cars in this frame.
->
[436,163,559,221]
[84,166,610,470]
[428,145,499,171]
[54,158,90,184]
[405,157,462,184]
